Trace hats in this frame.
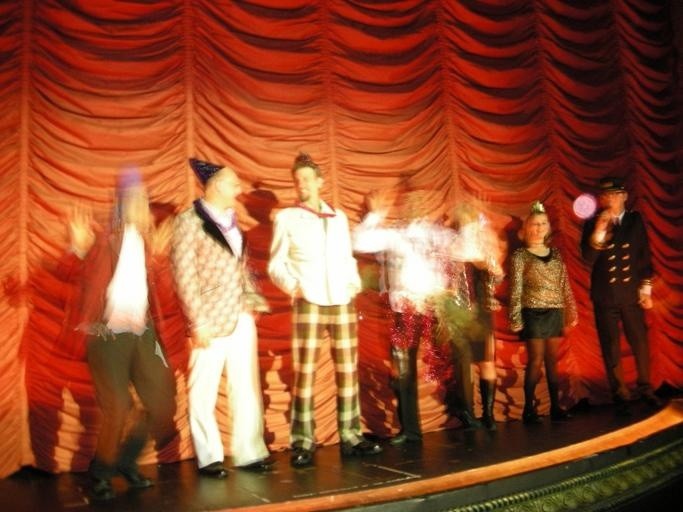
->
[187,156,225,185]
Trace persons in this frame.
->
[266,152,384,467]
[444,206,508,435]
[168,158,273,480]
[509,201,579,429]
[351,172,485,447]
[581,178,663,416]
[55,167,177,501]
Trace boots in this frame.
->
[549,383,573,421]
[479,378,498,433]
[523,385,544,425]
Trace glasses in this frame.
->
[599,191,624,197]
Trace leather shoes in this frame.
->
[201,462,229,479]
[92,477,114,501]
[290,451,313,467]
[342,440,382,456]
[240,459,274,473]
[390,435,422,447]
[117,466,154,489]
